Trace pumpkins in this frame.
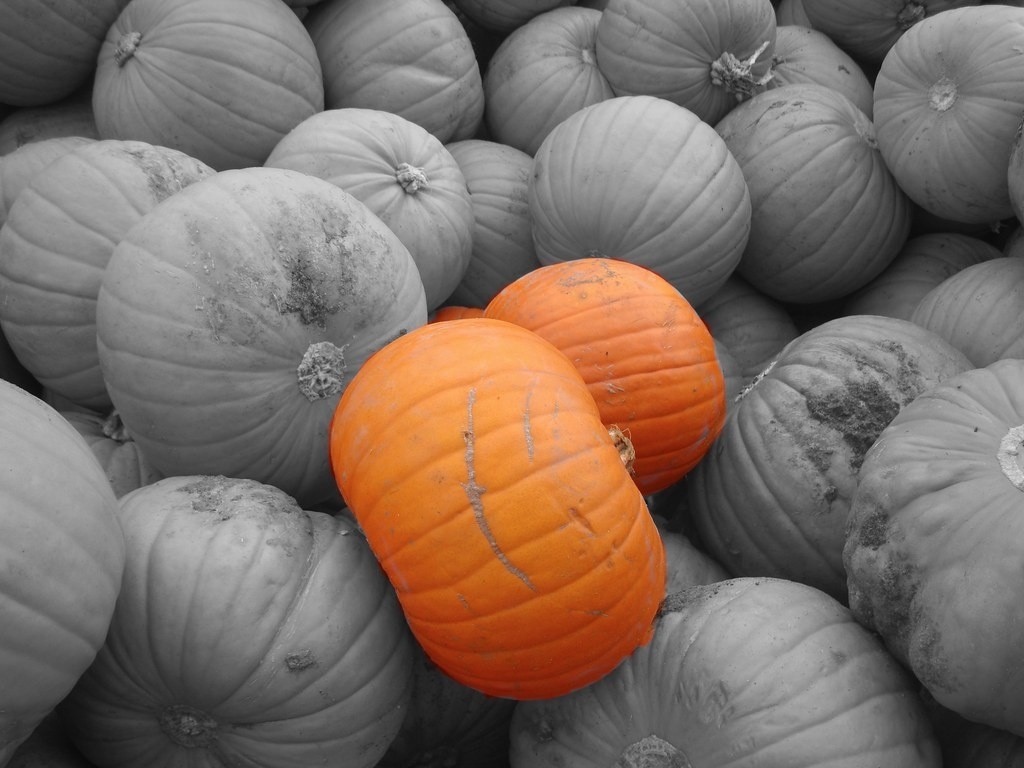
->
[1,0,1024,768]
